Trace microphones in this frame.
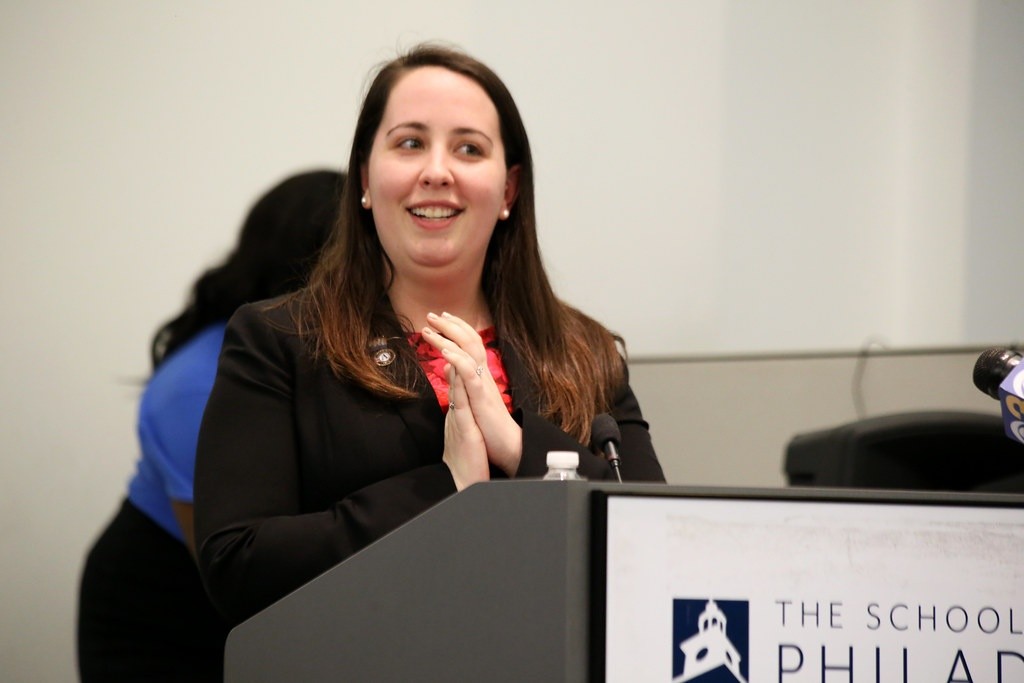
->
[589,412,628,484]
[971,343,1023,448]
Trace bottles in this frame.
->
[542,451,583,483]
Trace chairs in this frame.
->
[778,410,1024,492]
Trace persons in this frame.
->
[75,168,349,683]
[193,42,670,625]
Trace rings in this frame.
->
[476,366,484,376]
[449,400,455,409]
